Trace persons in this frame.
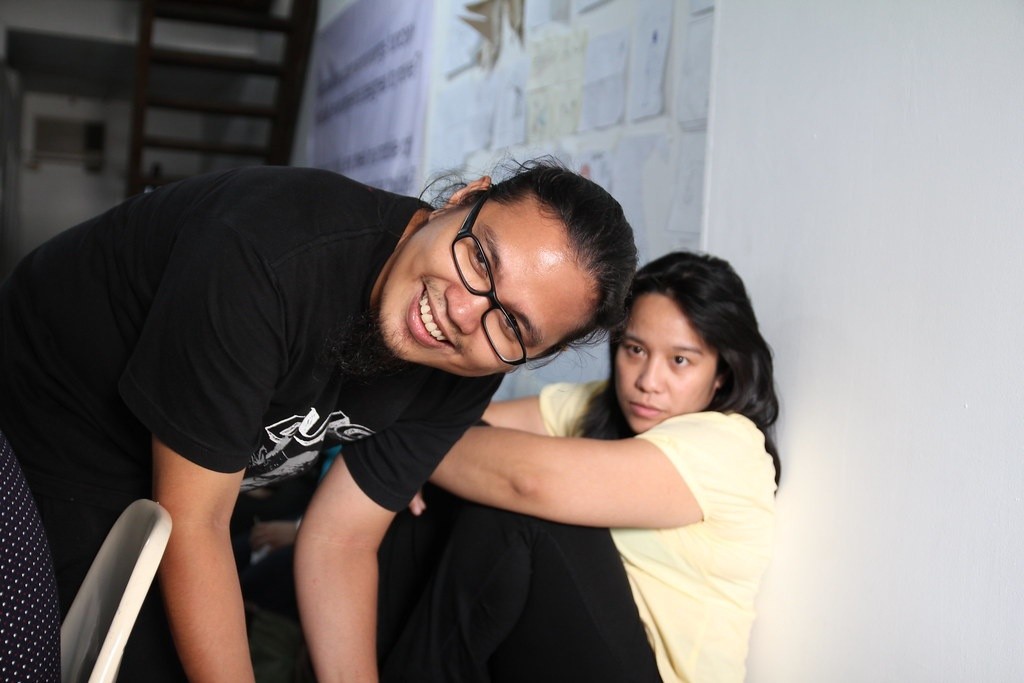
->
[0,154,782,683]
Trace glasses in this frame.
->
[450,189,564,365]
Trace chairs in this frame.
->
[61,499,174,683]
